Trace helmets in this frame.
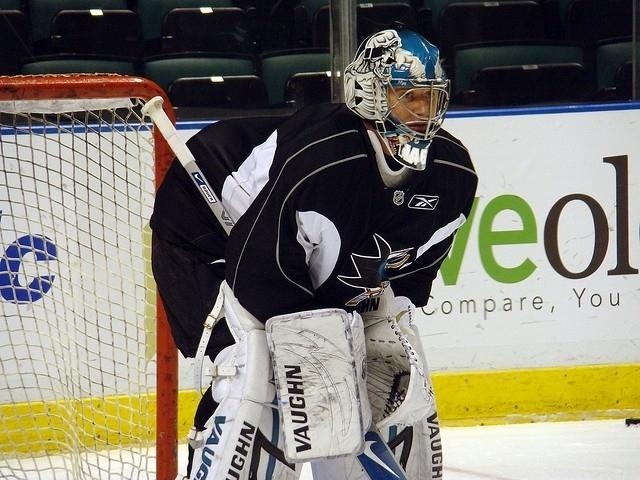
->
[343,29,460,172]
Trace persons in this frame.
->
[151,29,478,480]
